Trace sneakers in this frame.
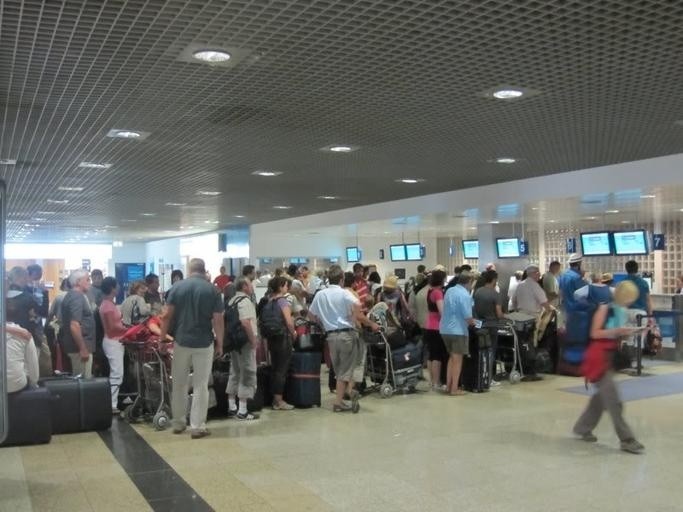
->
[351,389,360,413]
[334,401,351,412]
[620,439,645,453]
[192,428,211,438]
[271,401,294,411]
[227,407,259,420]
[573,427,597,442]
[427,381,466,396]
[174,426,187,434]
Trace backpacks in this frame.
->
[563,283,614,365]
[223,296,249,353]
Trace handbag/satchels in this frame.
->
[131,305,150,325]
[644,325,662,354]
[296,333,323,352]
[611,343,631,370]
[363,302,417,350]
[259,298,286,337]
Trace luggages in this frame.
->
[329,363,366,394]
[37,374,112,434]
[285,351,322,407]
[496,309,557,374]
[366,341,422,387]
[0,385,52,445]
[212,348,272,415]
[462,325,493,392]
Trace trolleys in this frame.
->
[115,334,179,432]
[359,326,424,397]
[491,315,544,385]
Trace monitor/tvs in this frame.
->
[330,256,338,263]
[390,244,405,261]
[580,231,612,257]
[642,277,652,290]
[358,251,361,259]
[299,257,309,263]
[346,247,358,262]
[462,239,479,259]
[496,237,521,259]
[420,247,425,257]
[612,229,648,256]
[262,258,272,264]
[289,257,299,264]
[405,243,420,261]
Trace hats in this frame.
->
[568,253,585,264]
[383,276,397,289]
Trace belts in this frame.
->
[327,328,354,333]
